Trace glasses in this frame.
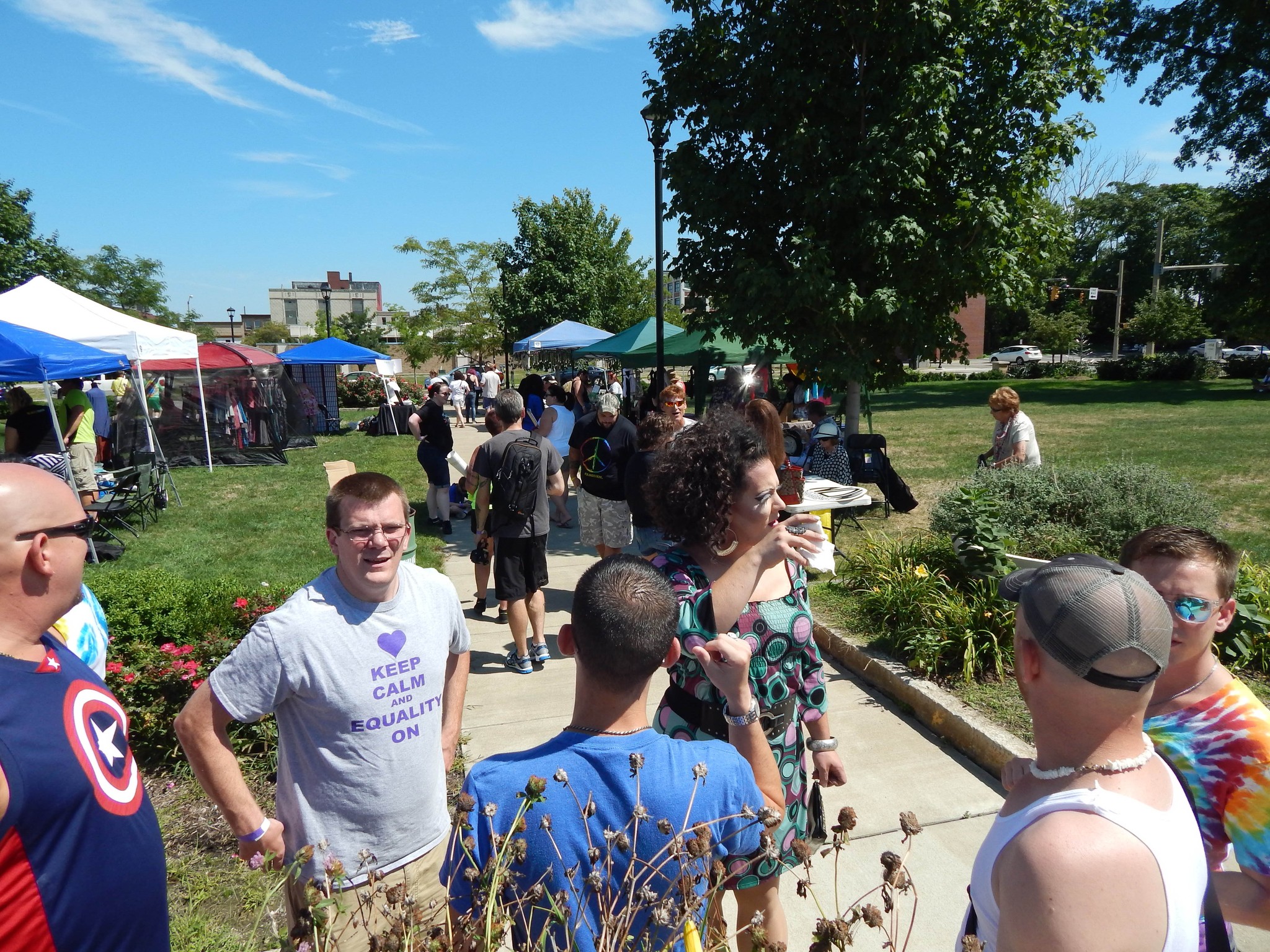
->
[460,487,467,493]
[1164,595,1226,624]
[15,514,96,540]
[331,522,411,541]
[990,406,1002,413]
[437,392,451,396]
[817,437,830,442]
[545,393,552,397]
[662,399,685,407]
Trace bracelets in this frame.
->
[806,737,839,752]
[237,817,270,842]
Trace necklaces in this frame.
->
[1149,652,1217,709]
[1029,731,1155,781]
[704,548,739,569]
[561,724,653,734]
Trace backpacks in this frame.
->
[489,435,543,548]
[561,379,574,393]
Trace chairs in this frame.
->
[131,450,162,510]
[84,472,140,548]
[844,434,890,517]
[318,404,344,435]
[90,462,159,531]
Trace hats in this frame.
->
[812,423,840,439]
[484,362,494,369]
[143,372,152,378]
[596,392,621,415]
[544,374,558,384]
[669,370,681,382]
[997,552,1174,693]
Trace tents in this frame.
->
[570,316,687,380]
[0,276,214,475]
[116,343,320,464]
[0,319,184,566]
[619,329,798,390]
[278,336,400,435]
[511,320,616,380]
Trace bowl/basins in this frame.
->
[403,401,412,406]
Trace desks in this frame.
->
[780,475,871,573]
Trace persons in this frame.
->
[472,389,565,674]
[0,461,172,952]
[439,554,784,951]
[568,392,638,559]
[143,373,164,419]
[809,423,854,514]
[1001,526,1270,952]
[57,379,99,530]
[659,385,701,452]
[407,382,454,535]
[744,398,786,471]
[111,371,134,405]
[429,363,811,434]
[465,408,509,624]
[449,476,472,520]
[173,471,472,952]
[625,412,675,561]
[531,385,575,529]
[284,375,320,434]
[977,387,1042,468]
[5,387,68,482]
[46,583,109,682]
[965,556,1209,951]
[640,402,846,952]
[790,401,842,472]
[85,382,112,460]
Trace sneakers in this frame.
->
[474,597,487,612]
[441,519,453,535]
[426,516,443,527]
[528,643,551,662]
[505,648,533,674]
[456,511,468,520]
[498,605,509,624]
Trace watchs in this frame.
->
[723,694,761,727]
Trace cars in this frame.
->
[425,365,504,388]
[1189,342,1269,362]
[990,345,1042,365]
[708,364,756,382]
[539,365,607,386]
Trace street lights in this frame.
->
[187,295,193,329]
[319,282,333,340]
[641,87,678,406]
[227,306,235,343]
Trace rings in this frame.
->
[785,525,807,536]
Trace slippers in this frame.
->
[549,516,573,527]
